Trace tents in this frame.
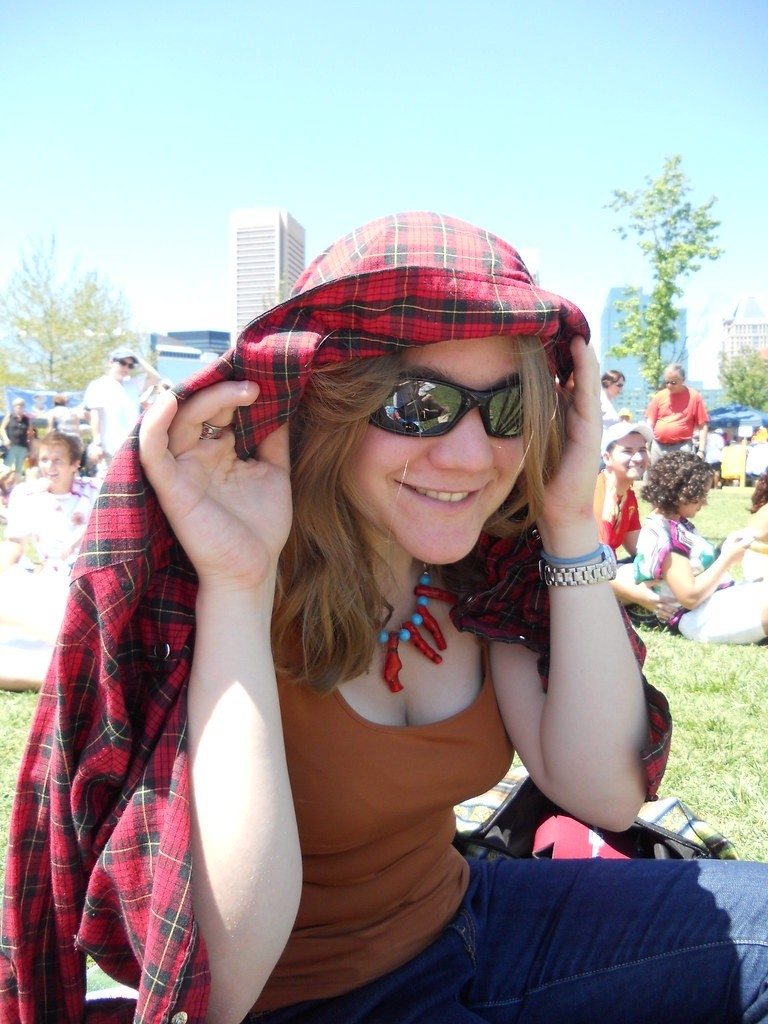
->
[703,402,768,428]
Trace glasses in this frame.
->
[118,359,133,369]
[613,380,623,388]
[665,380,677,385]
[369,379,557,439]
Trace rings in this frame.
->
[200,421,232,440]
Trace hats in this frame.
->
[618,408,633,421]
[111,347,139,365]
[601,422,653,456]
[714,428,724,434]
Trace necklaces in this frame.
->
[375,563,459,694]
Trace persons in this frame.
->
[1,213,768,1024]
[0,347,173,694]
[593,364,768,645]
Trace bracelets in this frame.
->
[537,546,617,587]
[540,544,604,565]
